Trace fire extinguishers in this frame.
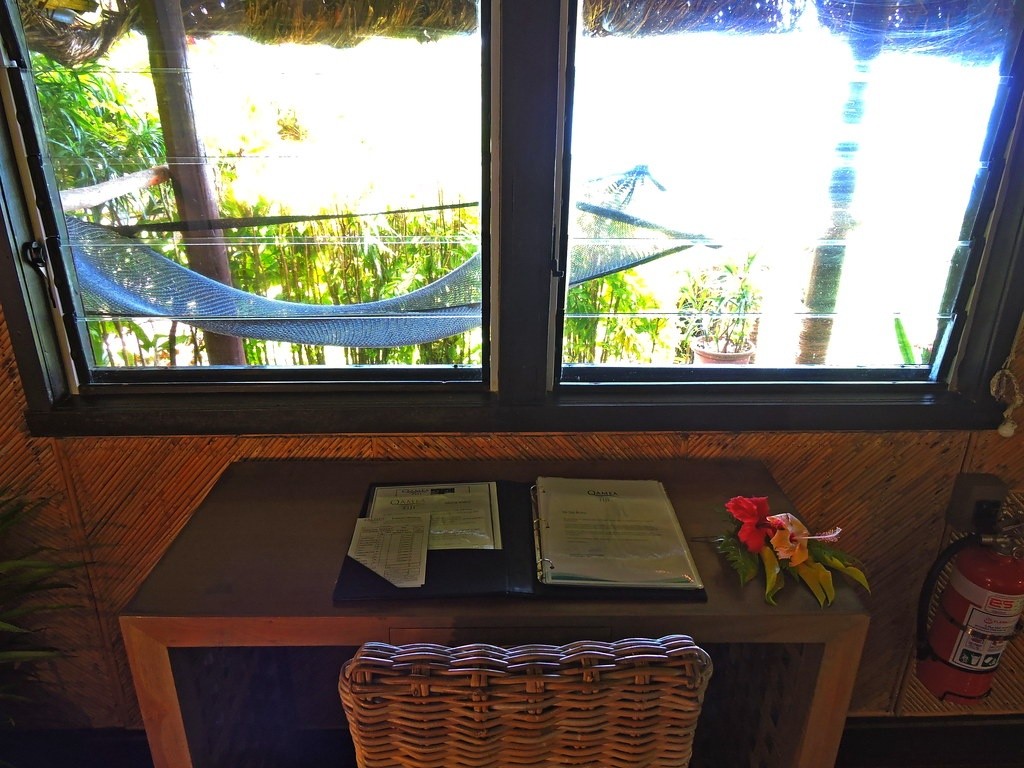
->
[915,515,1024,706]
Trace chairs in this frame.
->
[336,633,713,768]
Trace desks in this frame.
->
[118,455,873,768]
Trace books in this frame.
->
[333,476,707,606]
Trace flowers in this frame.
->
[691,496,872,609]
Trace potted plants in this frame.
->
[675,252,765,363]
